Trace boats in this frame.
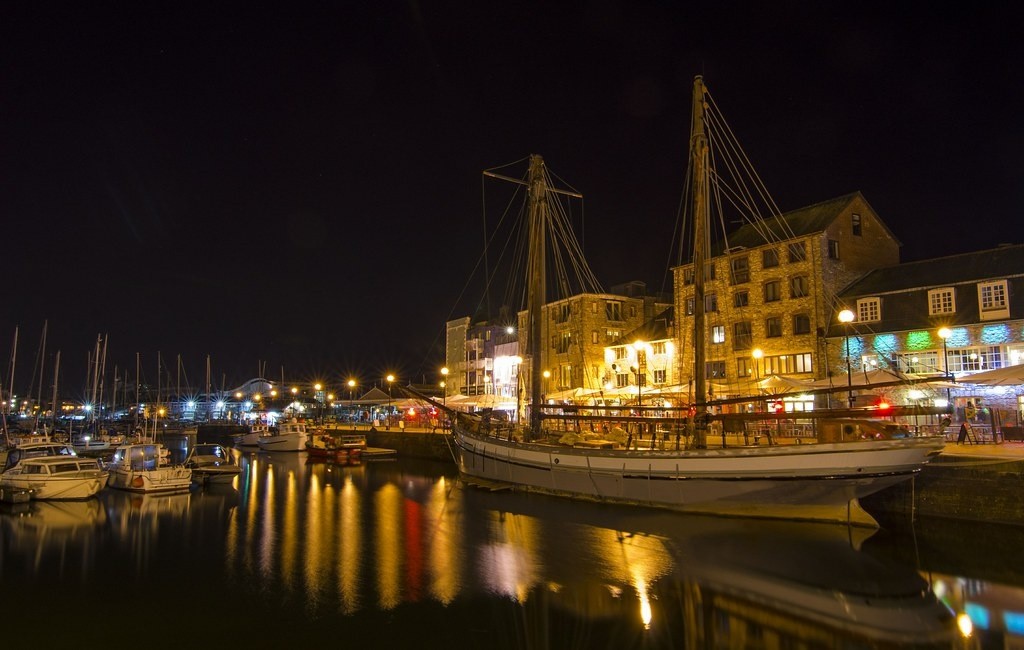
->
[257,420,310,451]
[103,443,192,491]
[0,435,111,503]
[174,444,243,484]
[304,429,397,462]
[230,420,272,445]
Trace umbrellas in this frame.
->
[546,368,966,403]
[955,364,1024,386]
[381,394,517,408]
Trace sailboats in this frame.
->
[0,319,198,454]
[394,75,957,529]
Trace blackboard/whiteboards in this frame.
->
[957,422,976,442]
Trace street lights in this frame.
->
[836,310,855,409]
[938,326,951,405]
[753,348,762,379]
[440,368,449,433]
[349,380,355,429]
[255,394,260,419]
[633,340,644,439]
[236,393,241,418]
[291,387,297,416]
[543,371,550,404]
[386,375,393,428]
[271,391,276,422]
[315,383,322,426]
